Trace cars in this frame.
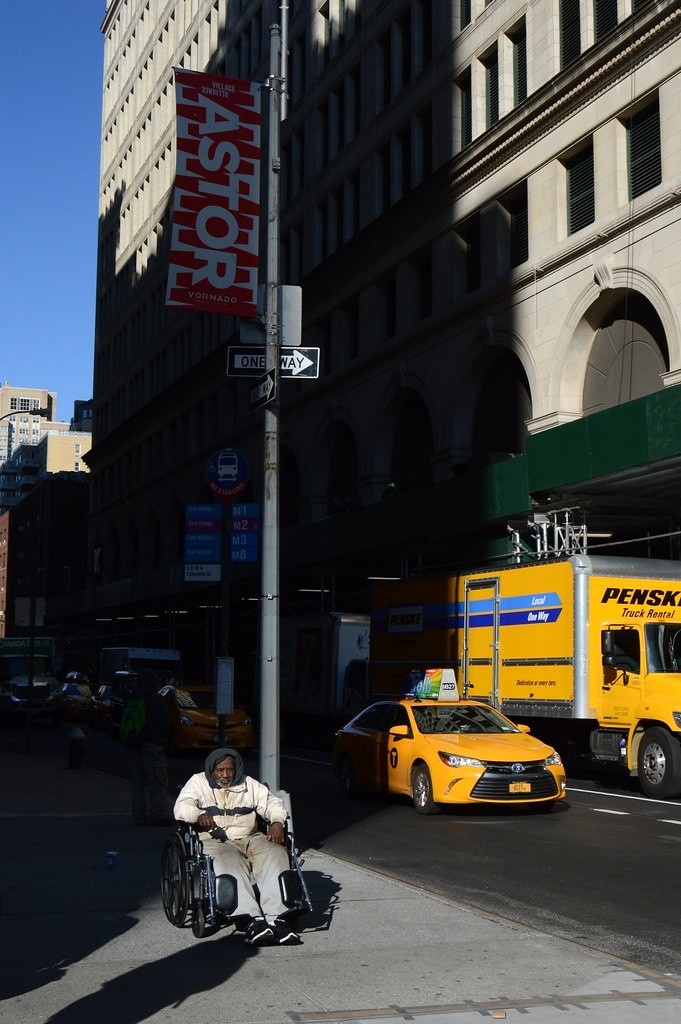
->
[51,668,257,762]
[330,668,569,814]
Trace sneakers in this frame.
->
[269,921,300,945]
[247,918,274,943]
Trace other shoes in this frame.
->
[136,818,147,826]
[150,818,172,827]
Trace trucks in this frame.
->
[368,552,681,799]
[0,637,56,724]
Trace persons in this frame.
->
[174,747,301,946]
[125,669,182,826]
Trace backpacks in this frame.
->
[118,691,157,749]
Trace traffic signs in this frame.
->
[246,366,276,416]
[227,345,322,380]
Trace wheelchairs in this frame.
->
[159,803,313,939]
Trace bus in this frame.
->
[96,647,184,696]
